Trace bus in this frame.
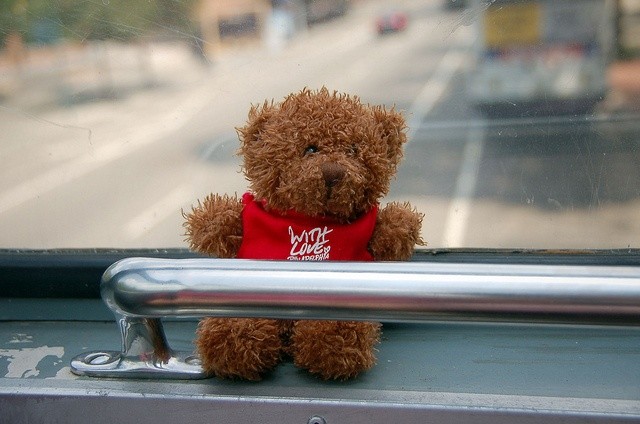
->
[466,0,615,107]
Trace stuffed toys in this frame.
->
[181,85,426,383]
